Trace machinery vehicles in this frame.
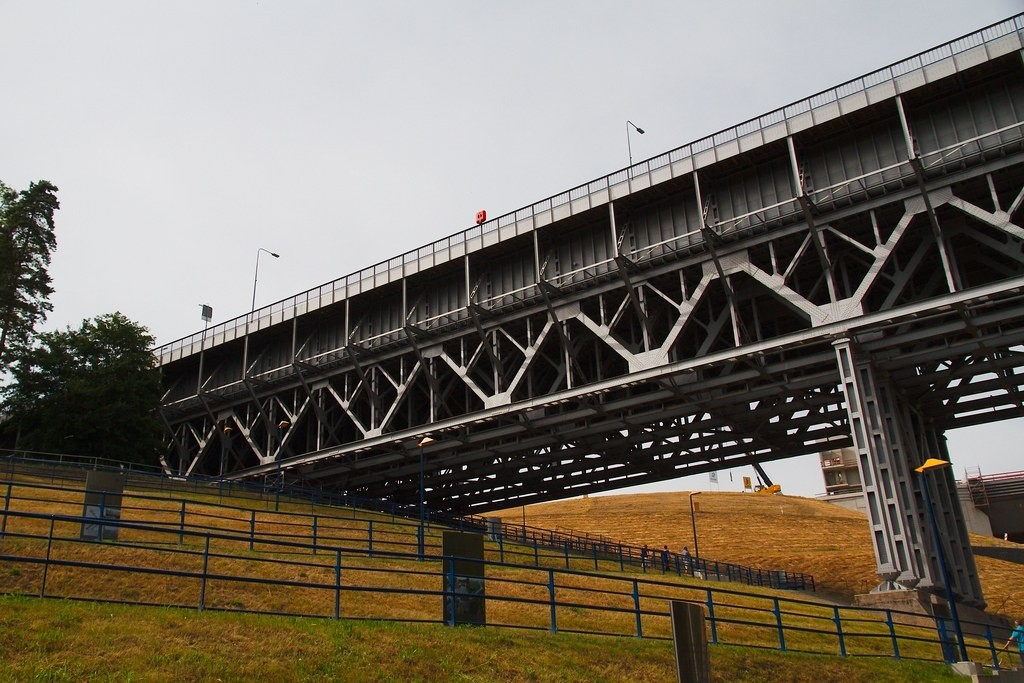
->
[727,426,782,496]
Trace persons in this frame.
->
[640,545,648,574]
[1003,619,1024,668]
[681,546,692,575]
[660,545,670,576]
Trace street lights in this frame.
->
[220,427,233,496]
[417,437,437,560]
[275,421,289,511]
[690,491,702,564]
[914,458,969,662]
[252,248,280,321]
[626,120,644,181]
